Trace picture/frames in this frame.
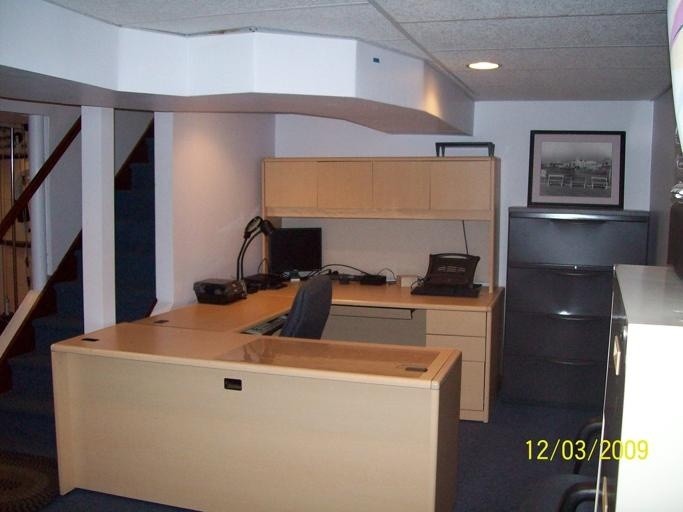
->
[527,129,626,211]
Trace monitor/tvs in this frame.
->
[263,227,322,282]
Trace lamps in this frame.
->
[236,215,273,293]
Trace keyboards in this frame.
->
[243,315,287,336]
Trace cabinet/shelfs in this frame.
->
[261,155,500,220]
[593,263,683,512]
[500,206,650,407]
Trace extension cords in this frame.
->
[326,272,354,281]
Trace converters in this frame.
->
[360,274,386,285]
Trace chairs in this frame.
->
[272,275,332,339]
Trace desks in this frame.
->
[52,279,506,512]
